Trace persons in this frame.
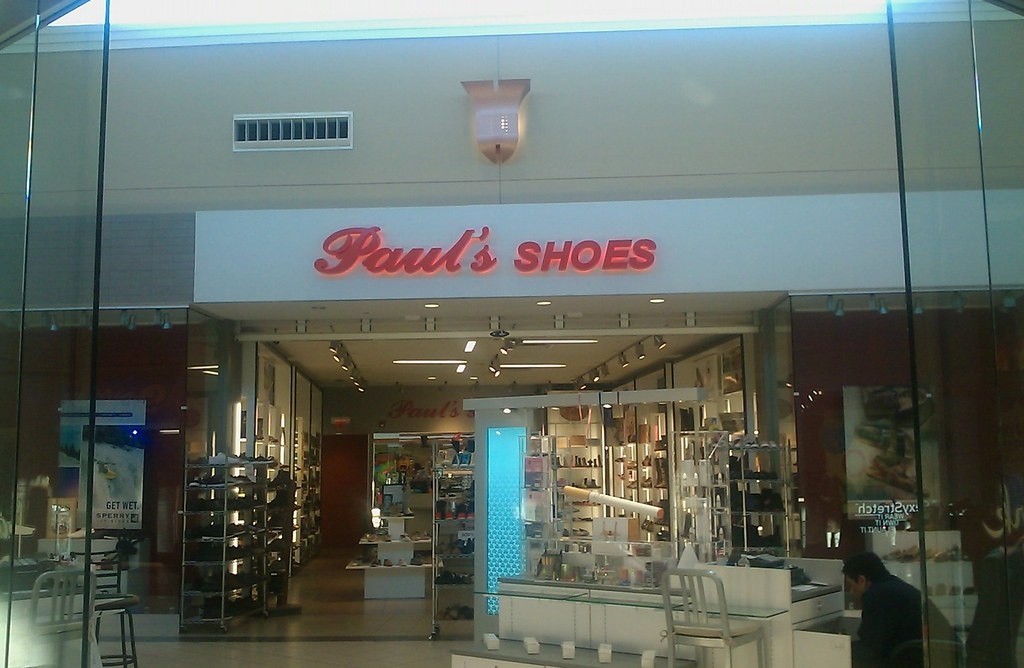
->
[841,550,961,668]
[966,529,1024,668]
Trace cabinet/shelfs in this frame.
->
[180,463,302,633]
[791,582,862,668]
[241,341,323,578]
[545,336,790,547]
[344,517,444,571]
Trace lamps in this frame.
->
[575,335,681,390]
[489,338,523,373]
[328,340,366,393]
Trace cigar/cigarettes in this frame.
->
[563,485,664,519]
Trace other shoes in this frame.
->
[185,523,265,541]
[208,452,274,466]
[435,474,476,519]
[411,532,430,541]
[398,558,406,566]
[438,539,474,555]
[384,558,393,566]
[399,534,410,542]
[350,556,367,566]
[438,440,475,468]
[267,469,290,572]
[384,535,391,542]
[371,558,382,567]
[189,497,255,511]
[185,546,251,562]
[191,573,269,592]
[202,597,259,622]
[360,532,380,542]
[188,475,253,487]
[411,559,423,565]
[435,570,472,584]
[437,605,474,620]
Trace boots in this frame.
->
[654,459,669,489]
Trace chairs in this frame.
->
[31,569,97,668]
[661,568,767,668]
[69,549,140,668]
